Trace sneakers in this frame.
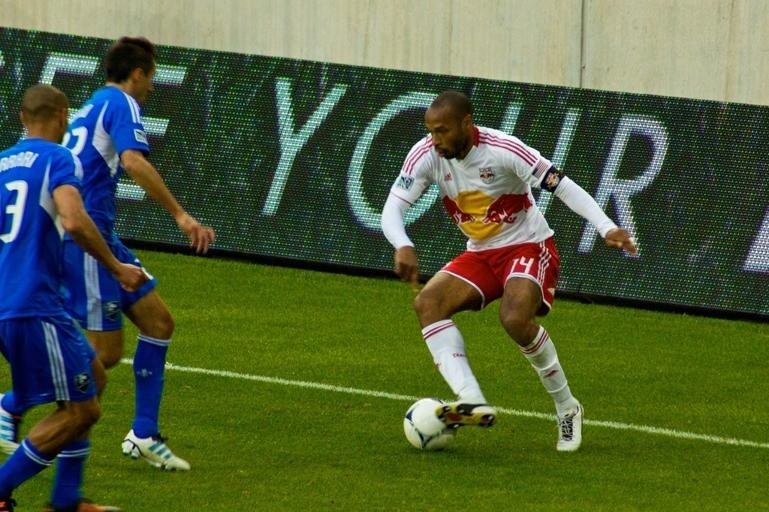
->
[121,430,192,472]
[445,402,497,427]
[0,497,13,512]
[43,498,122,512]
[553,402,584,451]
[0,394,22,454]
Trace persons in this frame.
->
[381,87,643,456]
[0,33,218,475]
[0,82,150,511]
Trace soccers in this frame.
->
[402,397,457,454]
